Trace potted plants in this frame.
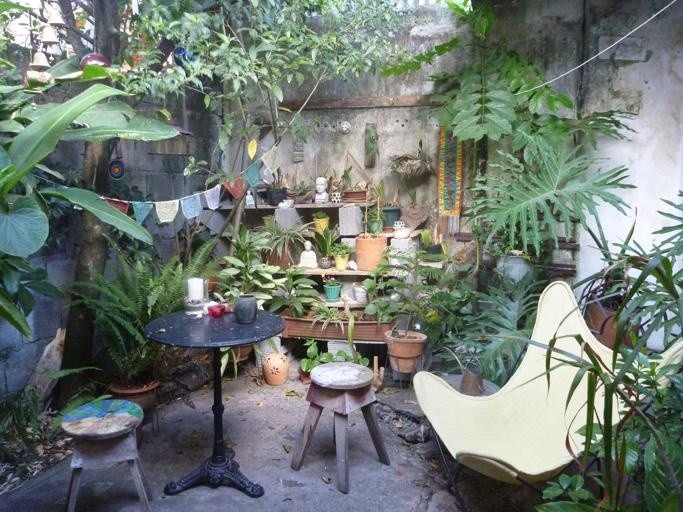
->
[42,235,217,423]
[491,219,549,290]
[214,124,443,386]
[571,207,667,354]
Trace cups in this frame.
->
[207,304,226,319]
[234,294,257,324]
[182,276,209,317]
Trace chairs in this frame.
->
[413,281,683,512]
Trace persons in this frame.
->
[299,240,318,268]
[315,175,329,203]
[245,190,254,205]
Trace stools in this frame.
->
[61,400,152,512]
[293,362,390,494]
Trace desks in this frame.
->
[142,310,285,498]
